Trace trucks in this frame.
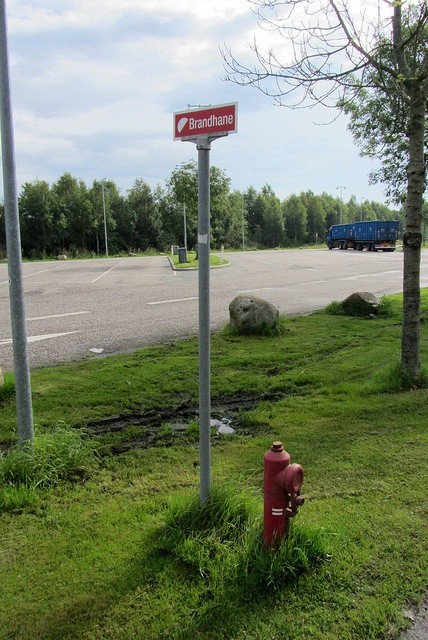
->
[325,221,398,251]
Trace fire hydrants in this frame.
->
[264,442,303,547]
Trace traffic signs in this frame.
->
[172,103,235,141]
